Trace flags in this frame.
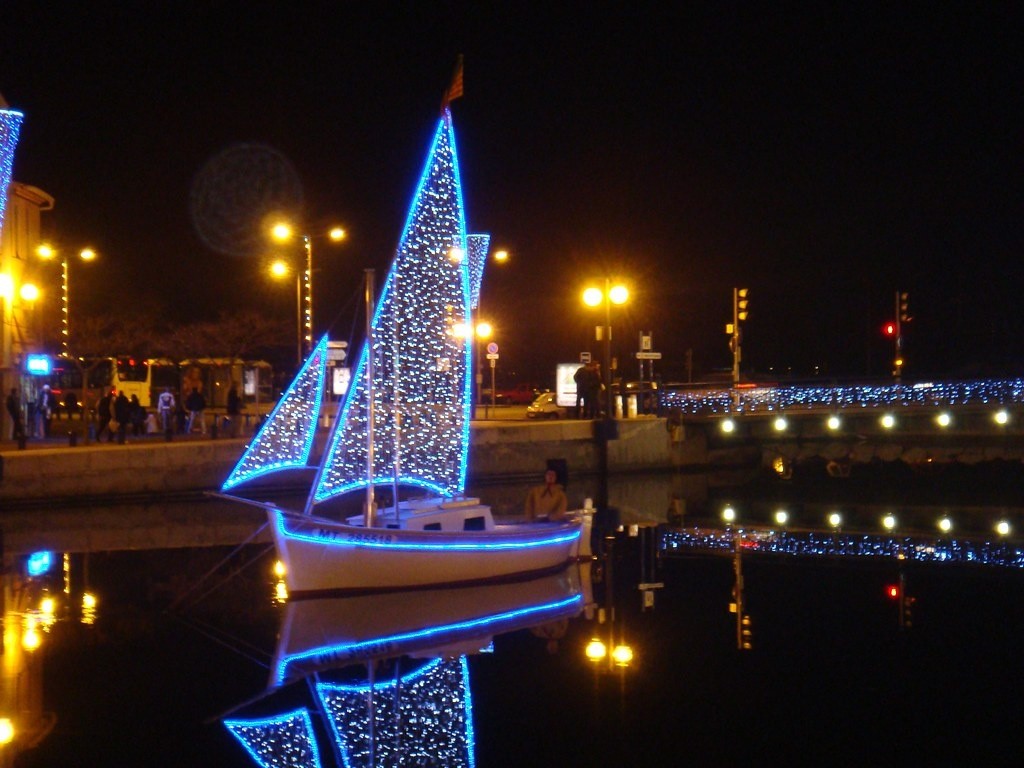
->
[441,65,463,123]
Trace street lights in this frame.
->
[33,240,98,358]
[578,270,631,413]
[22,551,98,649]
[266,211,348,383]
[445,246,506,418]
[582,522,634,670]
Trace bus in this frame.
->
[33,352,278,434]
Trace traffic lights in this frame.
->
[889,587,898,597]
[897,292,915,326]
[886,326,893,336]
[734,288,752,323]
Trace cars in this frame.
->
[526,391,567,421]
[495,381,539,403]
[605,379,666,411]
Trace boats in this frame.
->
[203,53,583,594]
[218,567,583,768]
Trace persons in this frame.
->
[186,387,206,433]
[574,361,605,419]
[533,388,541,400]
[35,384,56,438]
[525,470,567,523]
[6,388,27,440]
[227,381,247,439]
[95,388,189,445]
[183,367,203,395]
[65,391,78,421]
